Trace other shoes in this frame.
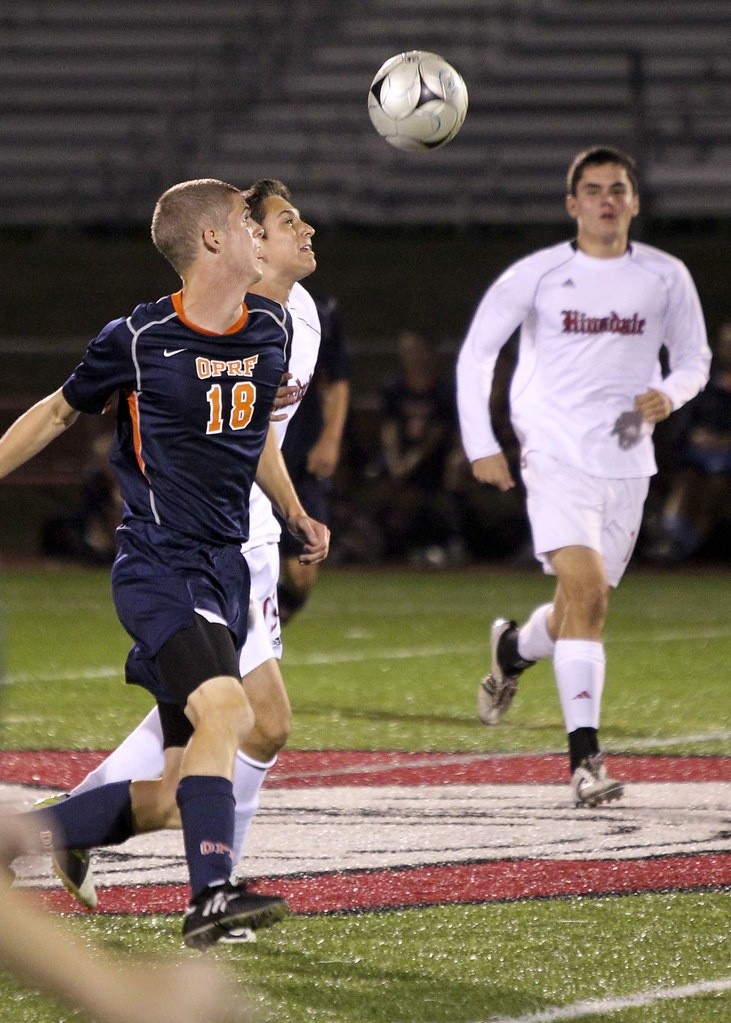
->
[184,906,257,945]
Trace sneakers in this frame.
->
[571,748,624,809]
[182,879,288,952]
[30,795,97,910]
[476,618,518,726]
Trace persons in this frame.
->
[0,178,362,1023]
[379,147,731,806]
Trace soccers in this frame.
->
[368,50,469,153]
